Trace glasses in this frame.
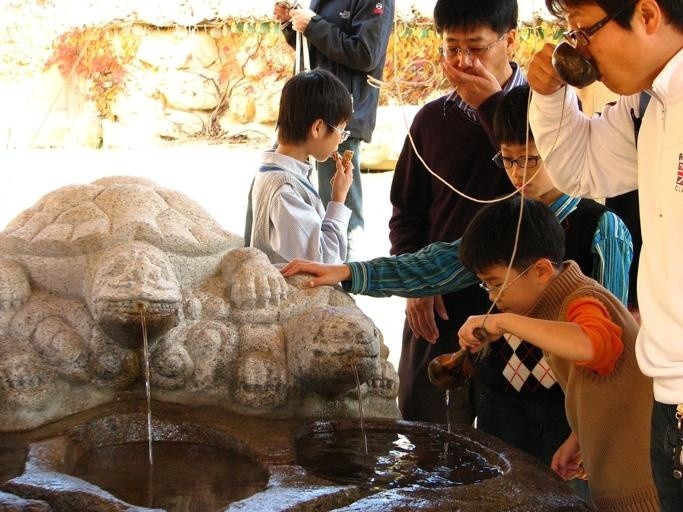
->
[563,7,623,46]
[438,35,505,56]
[328,123,351,142]
[492,151,541,167]
[479,264,534,297]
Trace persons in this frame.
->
[245,68,354,278]
[389,0,530,425]
[458,198,656,511]
[279,83,633,492]
[528,1,683,510]
[275,0,392,241]
